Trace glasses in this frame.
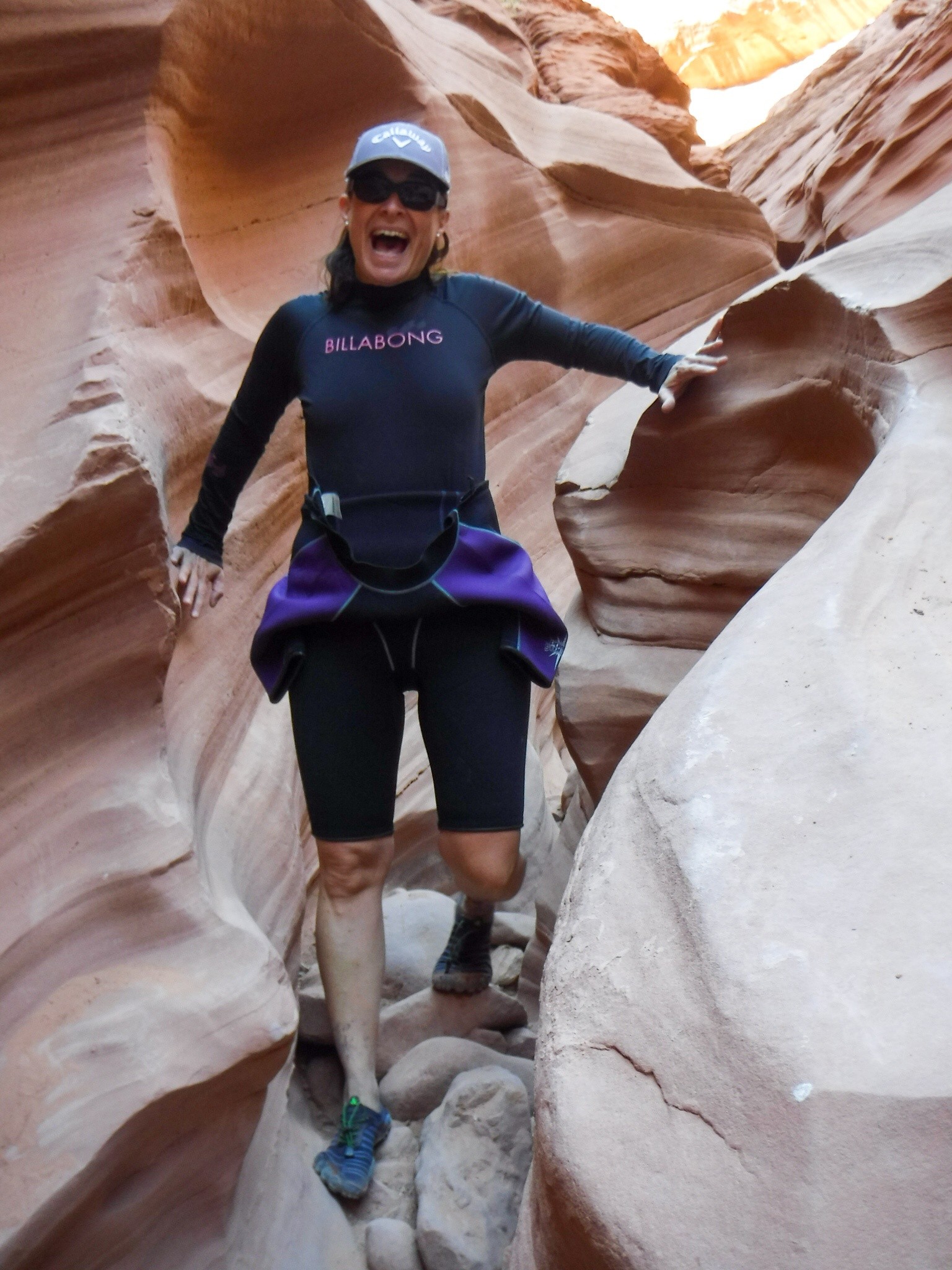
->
[354,170,446,212]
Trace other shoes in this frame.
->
[431,893,495,992]
[314,1096,392,1199]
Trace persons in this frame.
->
[170,120,730,1200]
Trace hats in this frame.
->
[346,122,451,190]
